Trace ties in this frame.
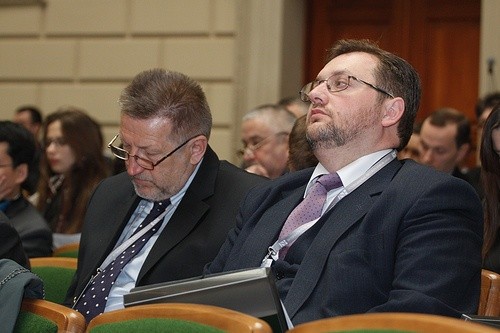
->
[279,172,343,261]
[74,199,171,321]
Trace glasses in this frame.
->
[237,131,291,158]
[106,134,207,170]
[299,73,395,104]
[45,138,67,146]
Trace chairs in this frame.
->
[28,258,79,304]
[84,301,274,333]
[10,297,87,333]
[286,310,500,333]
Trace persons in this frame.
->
[64,69,271,326]
[279,95,310,117]
[397,128,424,166]
[288,112,324,174]
[241,104,298,179]
[44,109,115,234]
[0,211,34,272]
[16,108,40,137]
[0,122,52,258]
[421,106,474,177]
[205,38,488,325]
[469,90,500,276]
